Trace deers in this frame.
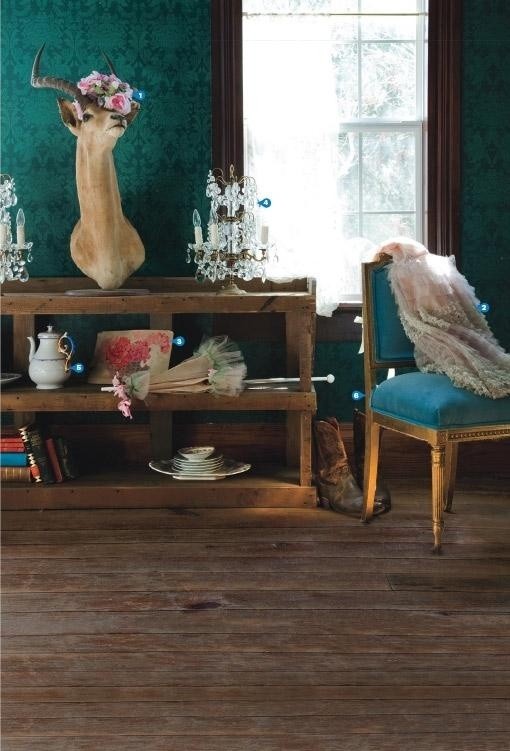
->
[27,43,147,291]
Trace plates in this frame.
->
[149,453,251,481]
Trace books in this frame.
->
[0,422,81,484]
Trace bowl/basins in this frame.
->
[178,446,215,460]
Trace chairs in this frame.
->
[359,259,510,550]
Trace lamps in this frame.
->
[184,163,278,295]
[0,170,34,287]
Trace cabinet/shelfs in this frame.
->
[1,273,319,510]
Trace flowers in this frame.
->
[73,70,135,121]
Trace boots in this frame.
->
[312,416,365,519]
[353,408,391,511]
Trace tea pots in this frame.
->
[27,325,77,389]
